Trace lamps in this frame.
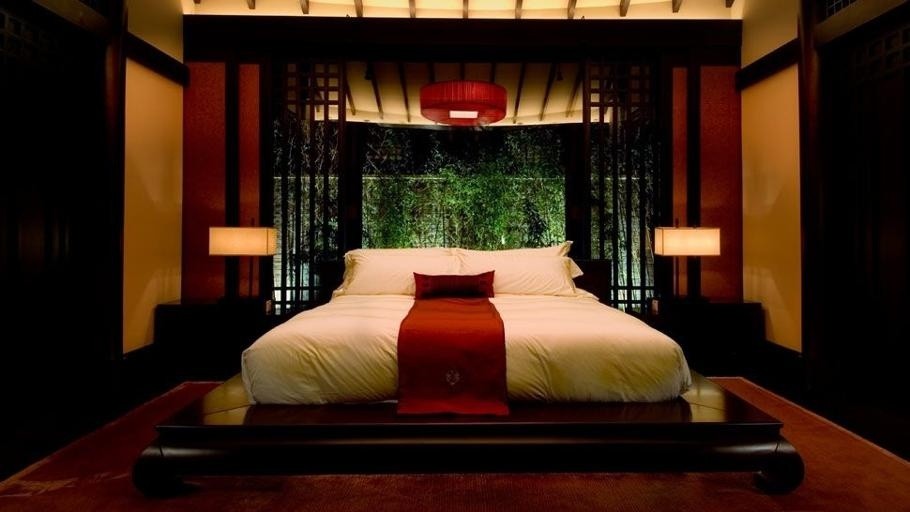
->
[655,227,720,306]
[208,226,277,299]
[419,78,509,126]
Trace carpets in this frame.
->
[0,376,909,512]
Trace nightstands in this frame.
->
[154,298,273,373]
[648,303,768,372]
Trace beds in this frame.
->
[240,247,693,406]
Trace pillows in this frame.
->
[332,240,578,297]
